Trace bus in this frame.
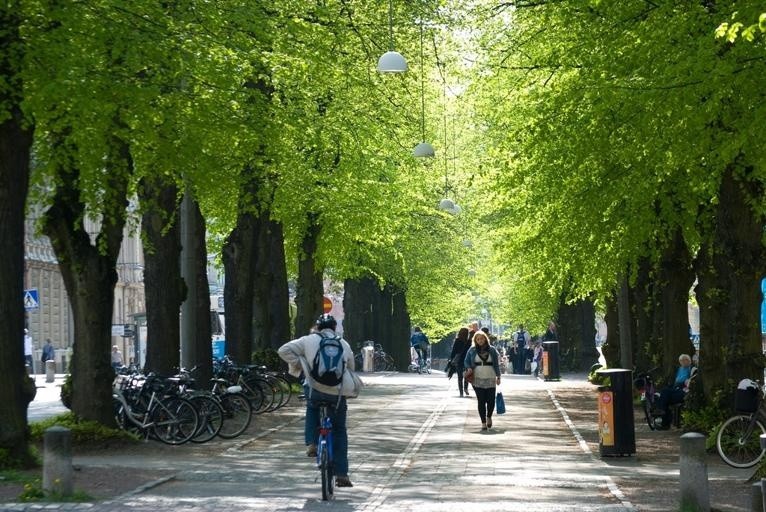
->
[208,293,297,364]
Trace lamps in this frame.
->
[376,0,487,315]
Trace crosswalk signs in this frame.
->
[22,288,39,309]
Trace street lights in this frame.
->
[120,281,129,366]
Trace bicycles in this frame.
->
[415,344,432,374]
[297,393,334,502]
[712,377,766,470]
[632,365,663,432]
[407,358,427,373]
[350,342,395,373]
[103,360,292,446]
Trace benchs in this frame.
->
[669,402,686,427]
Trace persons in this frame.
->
[277,314,362,486]
[41,339,54,362]
[412,327,429,365]
[652,353,698,430]
[444,321,556,397]
[464,331,500,429]
[24,328,35,374]
[111,345,123,367]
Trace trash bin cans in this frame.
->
[595,368,636,457]
[541,341,561,381]
[361,346,374,372]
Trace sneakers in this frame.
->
[304,444,318,458]
[459,388,469,398]
[336,472,353,488]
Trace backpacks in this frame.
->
[516,330,526,348]
[309,331,347,387]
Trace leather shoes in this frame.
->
[485,415,493,428]
[481,421,487,432]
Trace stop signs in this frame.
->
[322,295,332,316]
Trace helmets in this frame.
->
[314,312,337,330]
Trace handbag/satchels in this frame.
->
[463,369,473,384]
[496,378,506,414]
